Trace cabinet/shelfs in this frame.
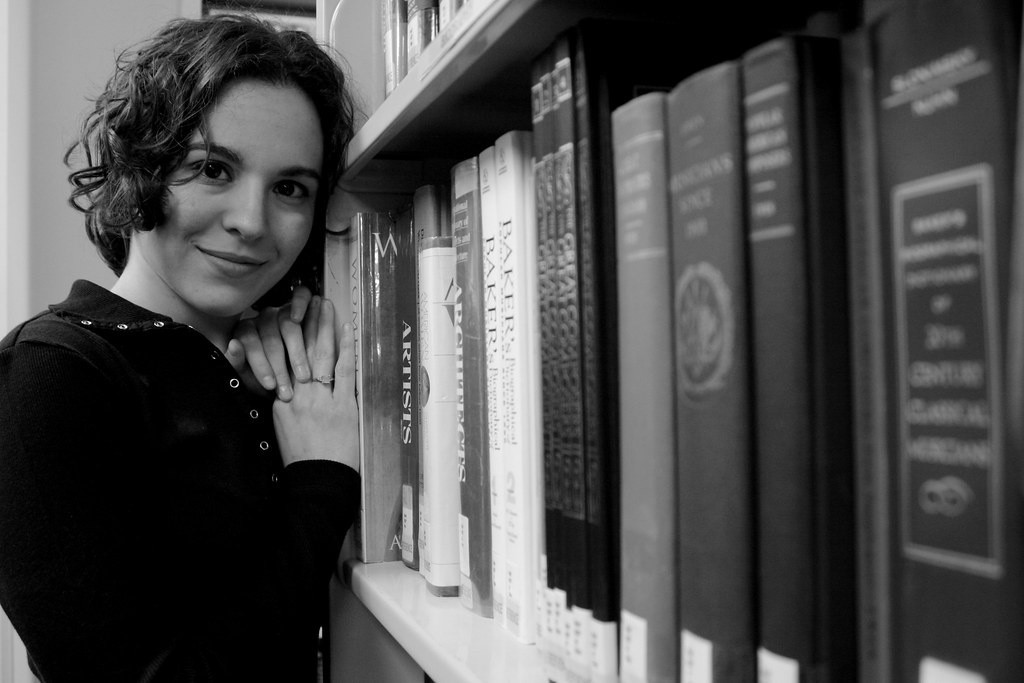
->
[315,0,599,683]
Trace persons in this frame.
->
[0,18,361,683]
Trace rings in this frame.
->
[311,374,334,384]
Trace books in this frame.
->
[335,0,1024,682]
